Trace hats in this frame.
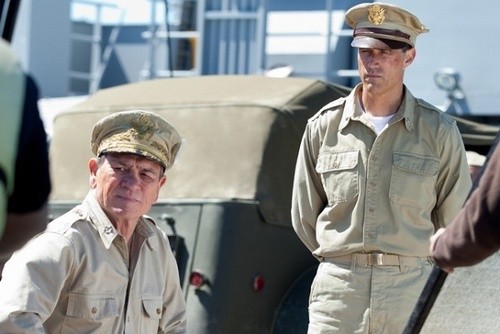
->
[90,110,183,171]
[345,3,430,50]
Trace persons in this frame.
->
[429,124,500,272]
[0,108,191,334]
[290,1,472,334]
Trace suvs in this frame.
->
[47,77,500,334]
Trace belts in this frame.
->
[324,252,434,269]
